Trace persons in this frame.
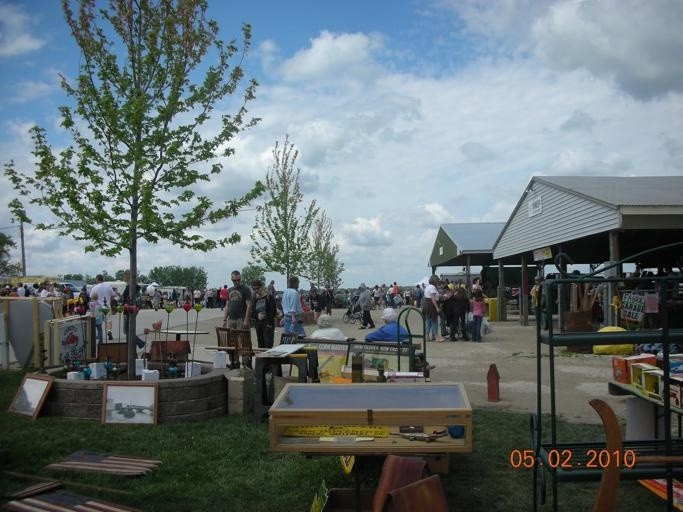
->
[0,270,490,380]
[530,276,545,329]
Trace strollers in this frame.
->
[342,294,364,325]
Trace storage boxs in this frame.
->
[266,382,473,454]
[316,485,392,512]
[610,351,682,409]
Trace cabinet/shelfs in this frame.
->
[533,271,682,512]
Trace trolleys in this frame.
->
[455,308,469,337]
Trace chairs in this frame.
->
[278,332,298,345]
[228,329,254,366]
[213,326,231,354]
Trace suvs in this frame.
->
[58,282,80,292]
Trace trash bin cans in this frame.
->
[488,298,497,321]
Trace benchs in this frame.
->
[403,342,436,383]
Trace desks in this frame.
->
[484,296,498,323]
[152,325,208,344]
[252,346,319,425]
[605,378,682,512]
[205,344,271,369]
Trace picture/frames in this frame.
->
[96,341,133,365]
[6,372,53,419]
[42,314,91,369]
[101,377,160,428]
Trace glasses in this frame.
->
[253,287,259,290]
[232,279,240,282]
